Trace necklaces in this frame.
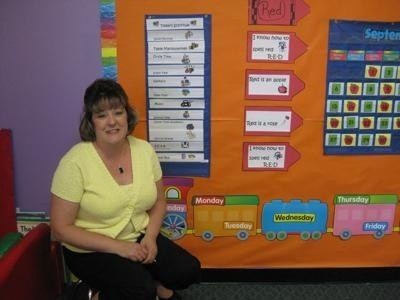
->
[95,138,125,174]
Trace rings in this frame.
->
[153,259,157,262]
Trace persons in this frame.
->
[51,79,200,299]
